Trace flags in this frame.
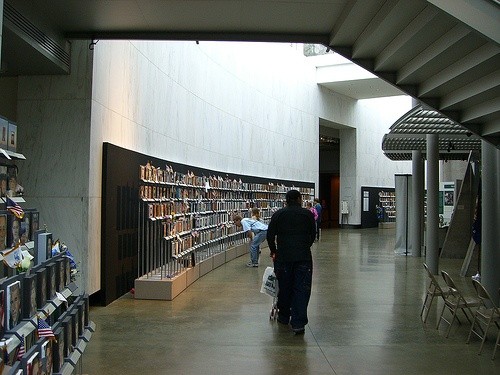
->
[16,335,25,360]
[37,317,55,338]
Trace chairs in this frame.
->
[436,270,488,342]
[419,262,462,326]
[464,279,500,356]
[491,288,500,360]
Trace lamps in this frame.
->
[448,140,454,153]
[465,131,475,138]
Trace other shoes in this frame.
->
[276,316,288,324]
[293,327,304,331]
[247,263,258,267]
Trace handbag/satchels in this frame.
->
[260,266,277,297]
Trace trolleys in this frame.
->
[268,252,278,322]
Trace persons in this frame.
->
[307,202,318,242]
[232,215,268,267]
[252,208,263,254]
[267,190,316,334]
[314,199,321,236]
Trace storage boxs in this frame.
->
[77,301,84,336]
[24,273,37,318]
[54,327,65,374]
[35,268,47,308]
[46,262,56,300]
[69,309,79,347]
[55,258,65,292]
[61,316,73,357]
[83,293,90,326]
[37,233,53,265]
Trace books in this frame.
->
[379,192,396,222]
[0,117,89,375]
[140,165,313,258]
